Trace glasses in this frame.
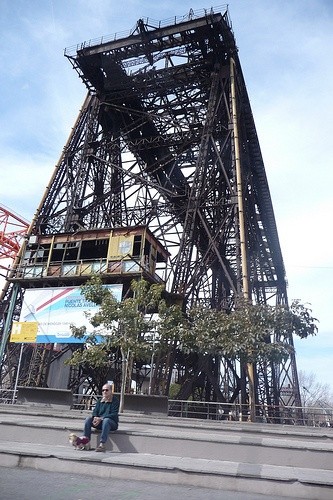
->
[102,389,109,392]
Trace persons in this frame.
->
[77,383,119,452]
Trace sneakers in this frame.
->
[95,442,105,452]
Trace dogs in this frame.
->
[68,434,90,450]
